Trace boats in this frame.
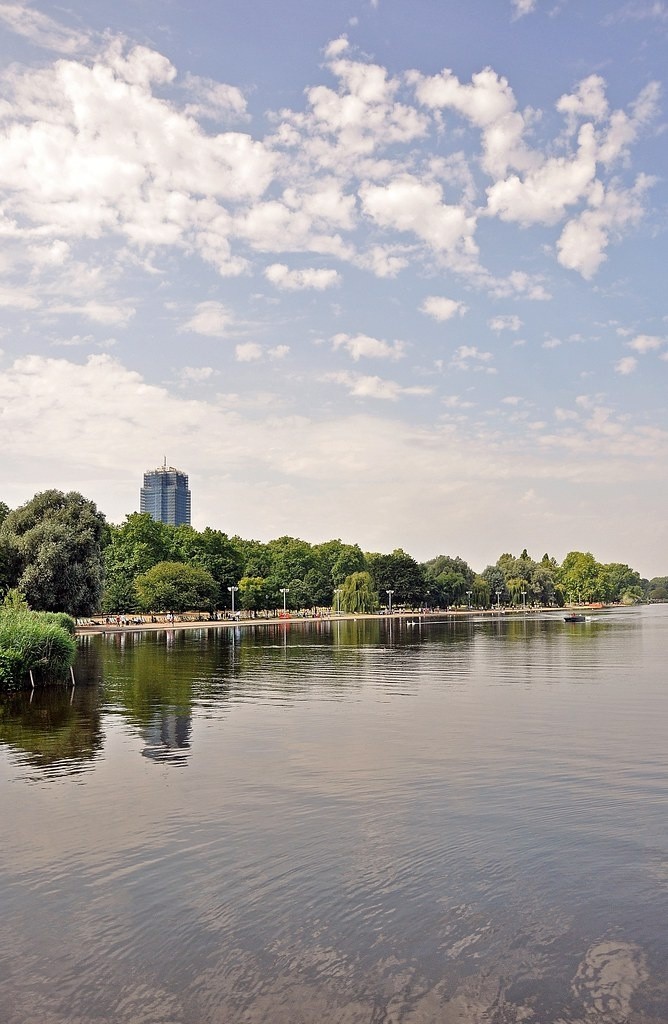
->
[564,616,585,622]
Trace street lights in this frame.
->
[465,592,473,608]
[495,592,502,609]
[228,586,238,612]
[279,588,290,612]
[386,590,394,611]
[334,589,343,614]
[521,592,527,607]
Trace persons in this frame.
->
[106,616,109,629]
[116,605,539,627]
